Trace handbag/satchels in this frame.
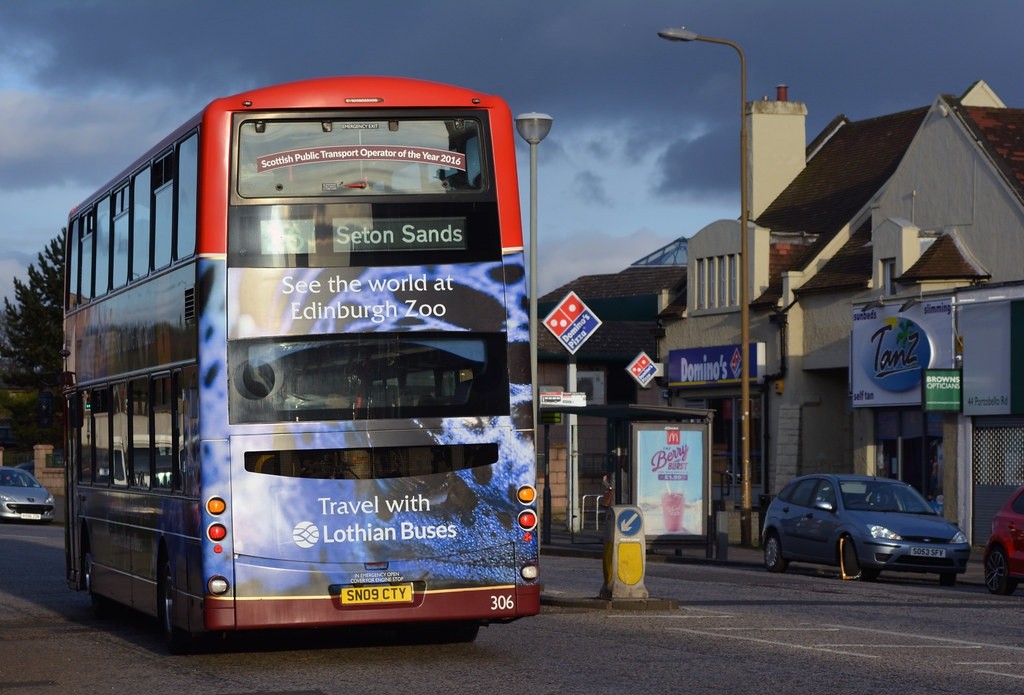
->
[601,485,613,508]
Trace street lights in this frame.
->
[515,109,554,447]
[655,23,753,548]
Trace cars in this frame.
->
[0,466,56,524]
[763,471,972,586]
[982,485,1024,597]
[13,450,63,478]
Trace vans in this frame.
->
[110,433,186,491]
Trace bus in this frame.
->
[37,75,545,655]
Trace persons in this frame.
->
[602,450,627,506]
